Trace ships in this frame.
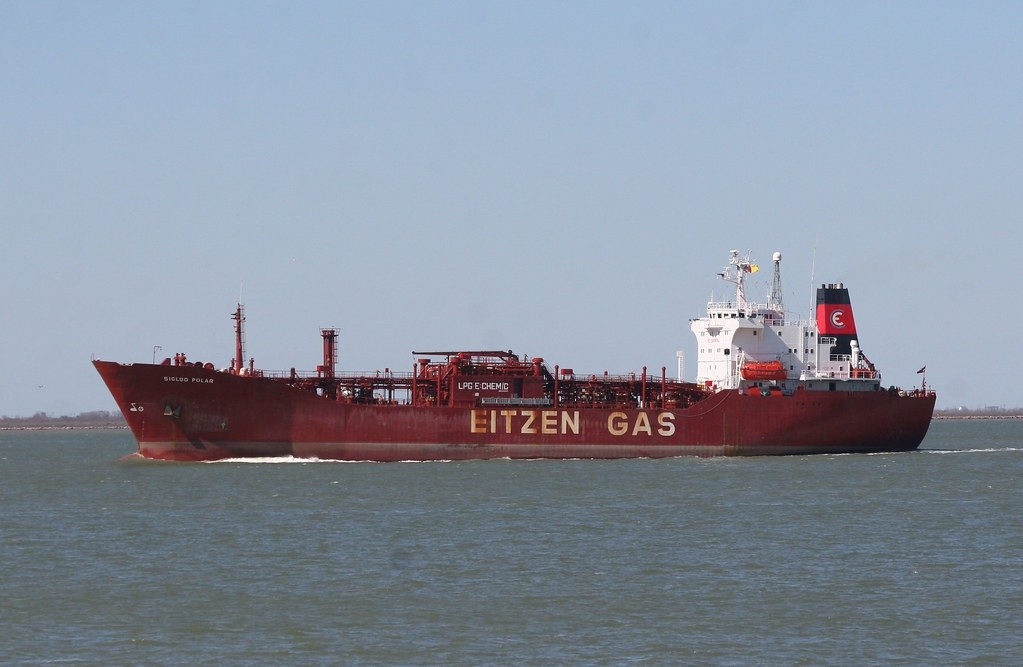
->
[90,250,936,462]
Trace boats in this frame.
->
[739,359,789,380]
